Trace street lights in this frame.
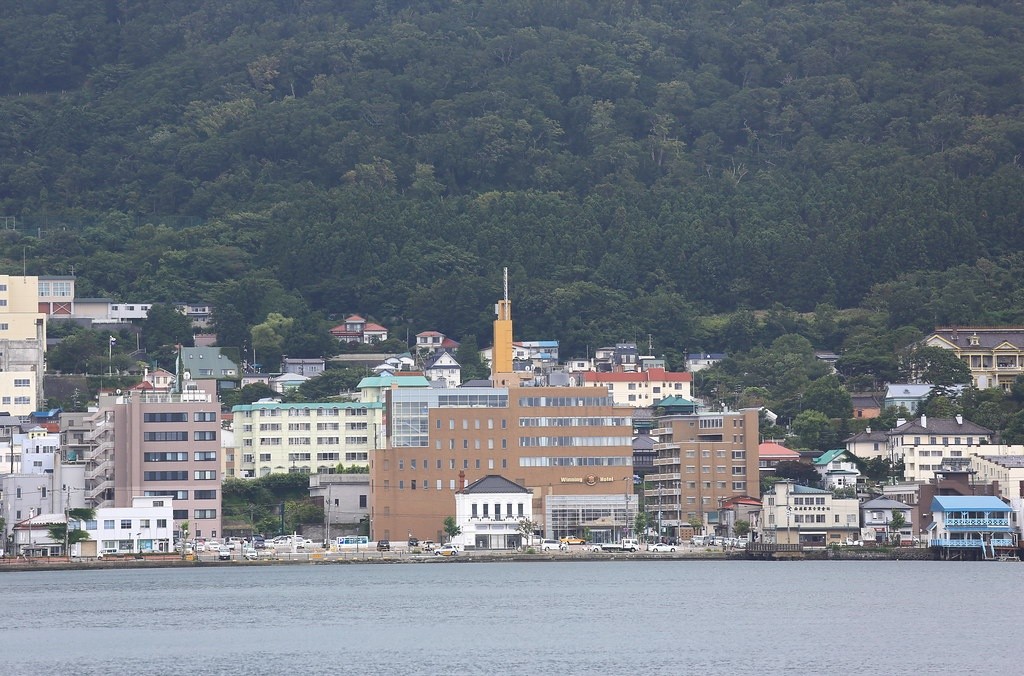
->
[655,482,665,542]
[672,480,682,546]
[540,524,544,552]
[623,476,631,540]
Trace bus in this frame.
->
[336,536,369,549]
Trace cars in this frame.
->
[433,543,459,557]
[407,538,419,547]
[704,535,749,548]
[648,542,678,553]
[377,539,390,552]
[175,534,323,557]
[245,549,258,560]
[560,536,586,545]
[421,541,436,552]
[591,543,605,552]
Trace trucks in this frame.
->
[601,539,641,553]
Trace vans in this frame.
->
[693,535,710,547]
[533,535,544,545]
[219,547,231,561]
[541,539,568,552]
[98,548,117,559]
